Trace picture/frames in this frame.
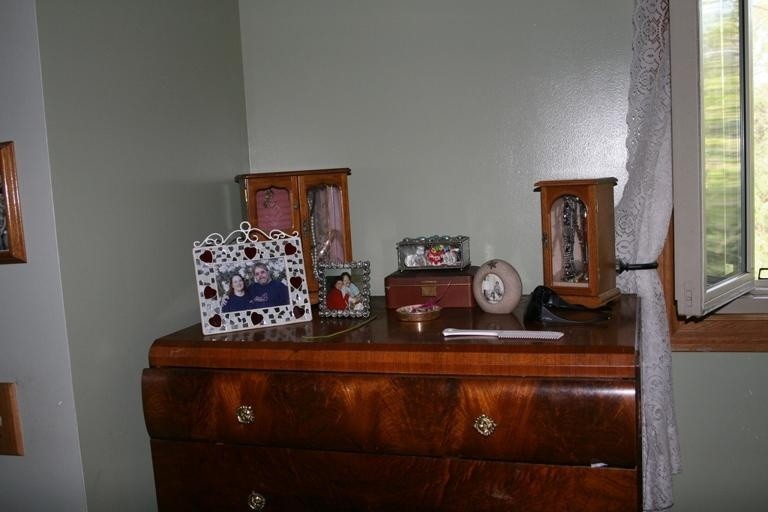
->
[191,222,313,338]
[317,260,372,320]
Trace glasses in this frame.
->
[522,285,612,329]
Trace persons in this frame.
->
[340,273,360,309]
[219,262,289,309]
[327,280,350,311]
[223,276,249,312]
[490,292,496,300]
[494,281,501,295]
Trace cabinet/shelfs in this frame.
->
[139,296,644,512]
